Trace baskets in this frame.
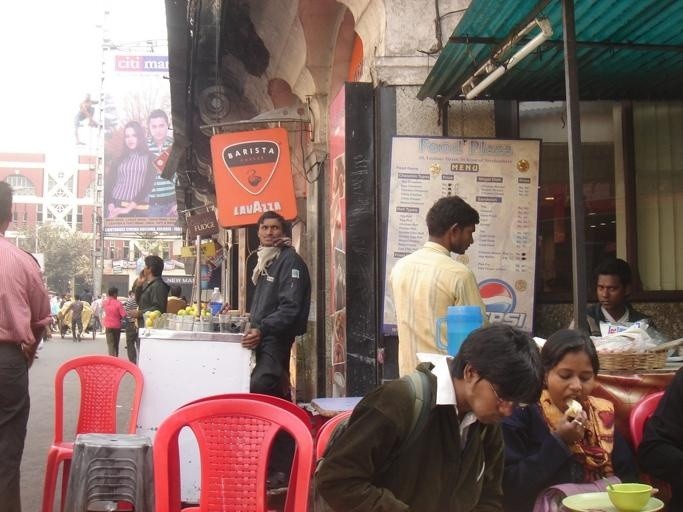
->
[594,337,682,376]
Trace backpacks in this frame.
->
[309,371,432,510]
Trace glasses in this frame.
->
[487,380,532,415]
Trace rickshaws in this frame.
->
[57,301,96,340]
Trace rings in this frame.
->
[583,426,586,429]
[574,420,582,426]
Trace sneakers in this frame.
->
[60,334,82,342]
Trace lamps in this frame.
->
[462,15,553,101]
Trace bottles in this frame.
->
[210,286,225,315]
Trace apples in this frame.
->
[178,303,211,320]
[144,310,161,327]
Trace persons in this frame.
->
[567,259,657,336]
[635,367,683,512]
[390,195,490,379]
[0,181,53,512]
[50,256,187,367]
[105,121,157,219]
[145,109,178,218]
[241,211,312,494]
[499,329,640,512]
[311,324,544,512]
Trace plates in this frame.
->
[559,491,665,511]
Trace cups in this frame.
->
[606,482,654,511]
[142,309,251,335]
[434,305,483,358]
